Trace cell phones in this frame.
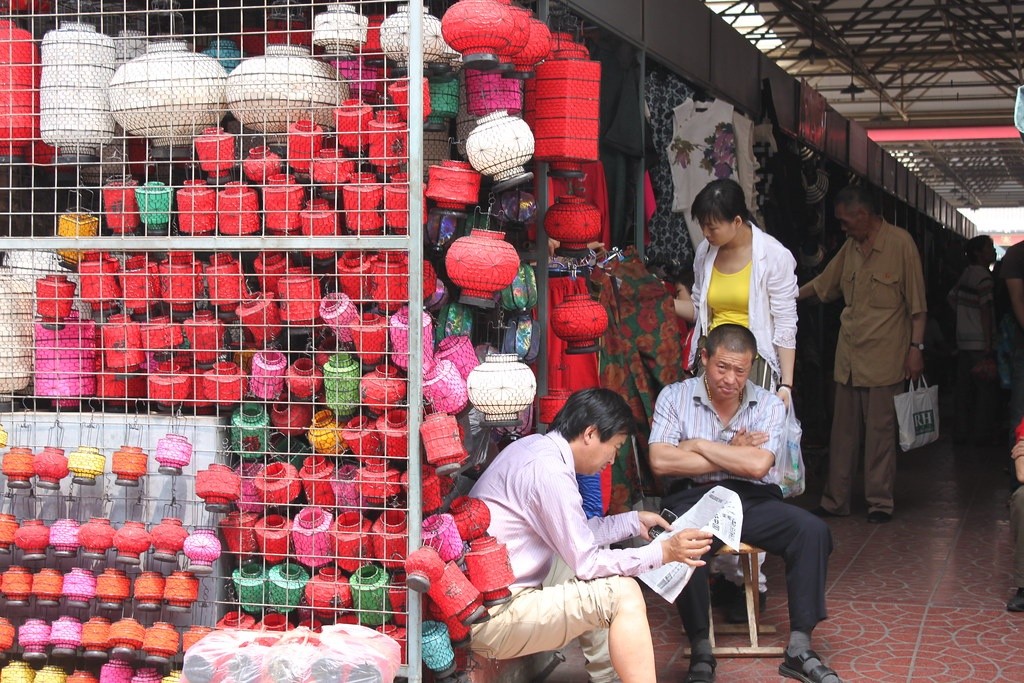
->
[648,508,677,539]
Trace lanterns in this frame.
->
[403,411,517,683]
[379,0,609,422]
[0,249,409,683]
[0,0,410,236]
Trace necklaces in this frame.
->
[705,375,742,406]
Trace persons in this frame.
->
[674,179,799,623]
[798,186,928,524]
[676,270,696,370]
[946,235,1024,612]
[470,387,714,683]
[647,323,842,683]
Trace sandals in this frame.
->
[778,644,843,683]
[684,653,717,683]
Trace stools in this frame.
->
[681,542,785,658]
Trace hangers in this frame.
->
[548,245,626,279]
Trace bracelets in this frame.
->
[780,384,792,390]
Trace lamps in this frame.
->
[870,91,892,127]
[937,172,979,213]
[797,15,827,66]
[839,60,866,102]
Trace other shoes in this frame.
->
[867,511,890,524]
[809,506,831,517]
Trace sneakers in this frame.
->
[1006,586,1024,613]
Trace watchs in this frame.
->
[911,343,926,350]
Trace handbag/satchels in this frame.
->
[893,375,941,453]
[766,386,806,500]
[970,358,998,388]
